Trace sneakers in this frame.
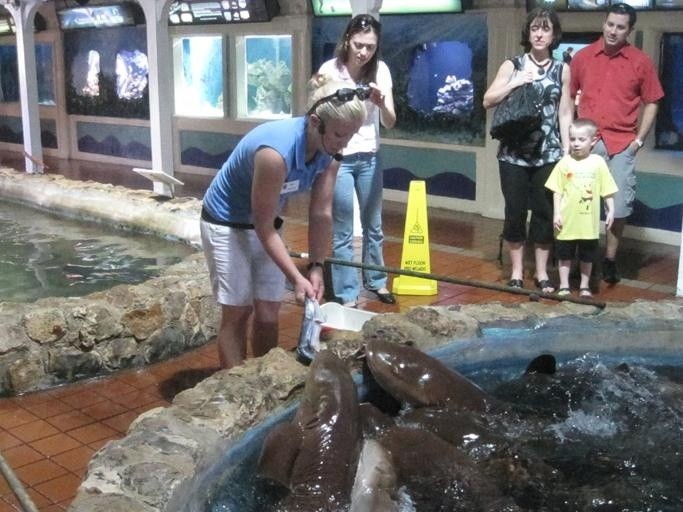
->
[602,257,621,283]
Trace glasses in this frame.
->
[352,19,381,32]
[308,88,371,115]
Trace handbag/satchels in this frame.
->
[490,84,544,145]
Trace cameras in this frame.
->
[357,84,371,95]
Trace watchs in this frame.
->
[633,137,646,148]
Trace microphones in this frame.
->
[318,124,343,161]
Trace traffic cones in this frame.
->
[386,175,438,296]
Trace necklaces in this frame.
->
[527,56,552,76]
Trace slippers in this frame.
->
[535,278,555,293]
[508,280,523,288]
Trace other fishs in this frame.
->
[253,340,683,510]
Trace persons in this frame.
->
[481,6,573,294]
[199,82,367,369]
[568,2,666,294]
[543,117,620,300]
[561,45,573,64]
[304,13,395,311]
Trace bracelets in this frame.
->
[304,262,330,276]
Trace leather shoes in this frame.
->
[378,293,396,303]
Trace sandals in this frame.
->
[555,287,573,304]
[578,286,593,298]
